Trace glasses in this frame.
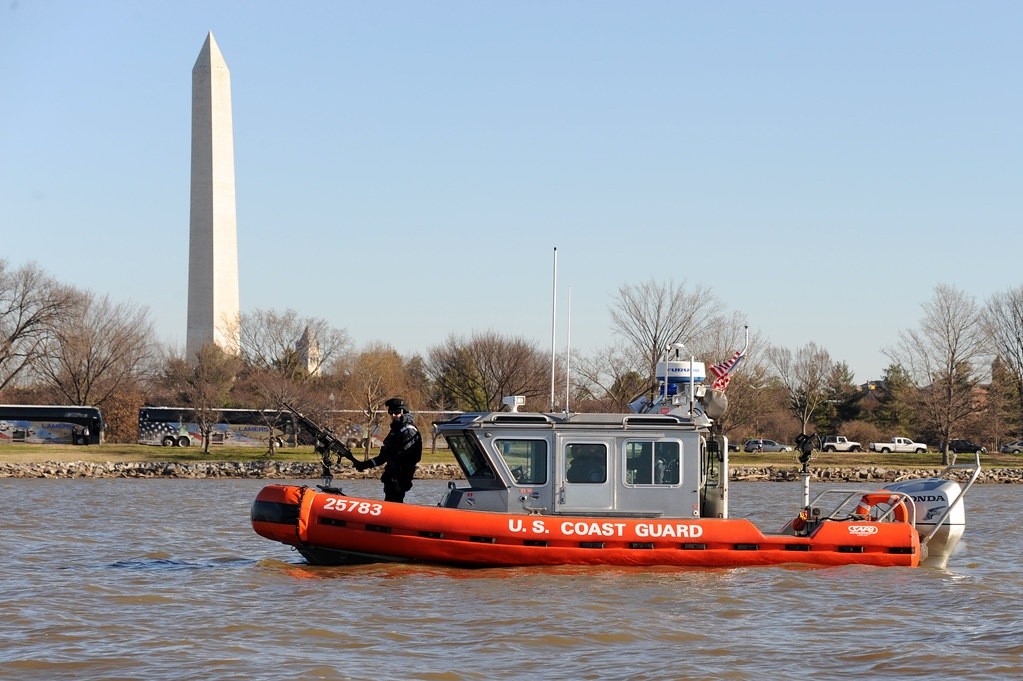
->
[388,409,401,414]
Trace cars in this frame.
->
[744,438,792,453]
[1000,440,1023,455]
[705,440,740,452]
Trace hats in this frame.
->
[385,398,409,414]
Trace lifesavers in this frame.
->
[856,489,910,523]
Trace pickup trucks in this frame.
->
[869,436,929,454]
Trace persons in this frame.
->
[627,443,664,484]
[72,426,78,445]
[352,398,423,503]
[566,446,601,481]
[82,426,89,445]
[754,437,764,456]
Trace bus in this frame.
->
[315,409,465,450]
[137,406,301,449]
[0,403,108,445]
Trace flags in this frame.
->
[710,352,741,391]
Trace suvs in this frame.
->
[817,433,863,453]
[938,438,988,455]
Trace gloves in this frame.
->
[352,458,376,472]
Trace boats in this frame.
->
[251,246,989,572]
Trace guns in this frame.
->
[283,401,359,464]
